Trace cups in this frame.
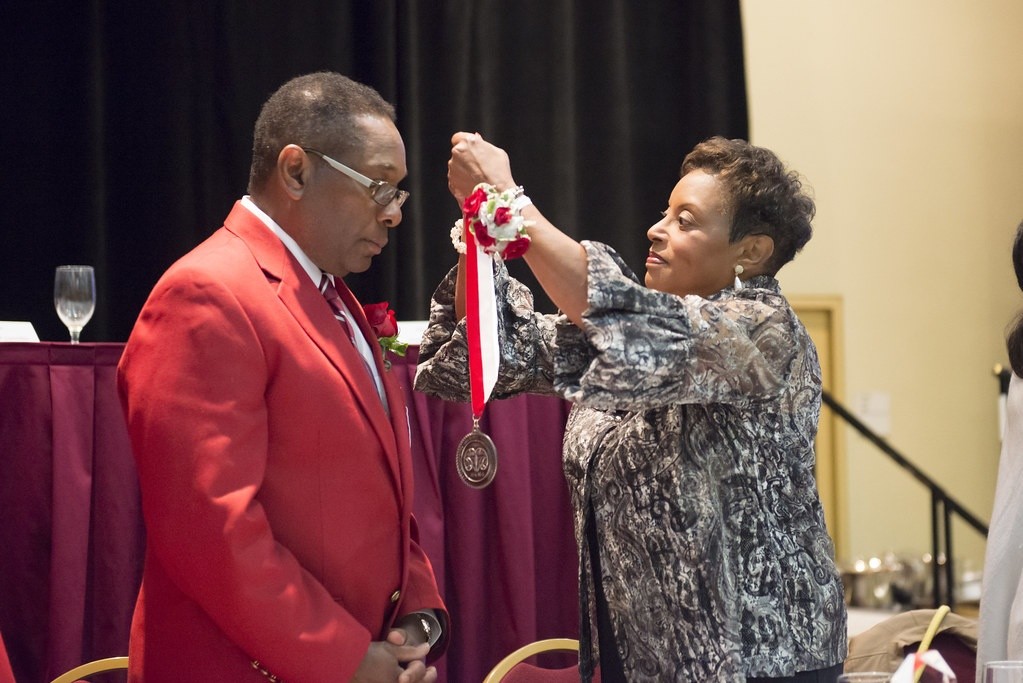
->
[985,661,1022,682]
[837,671,892,682]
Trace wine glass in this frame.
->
[54,266,96,343]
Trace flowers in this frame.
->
[464,183,540,263]
[364,301,411,371]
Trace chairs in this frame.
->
[844,607,978,683]
[48,656,130,683]
[479,638,600,683]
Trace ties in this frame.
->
[317,274,380,399]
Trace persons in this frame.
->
[974,220,1023,682]
[439,131,850,683]
[114,72,444,683]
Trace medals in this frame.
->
[455,414,498,491]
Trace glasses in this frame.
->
[303,148,410,208]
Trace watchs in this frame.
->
[415,612,432,642]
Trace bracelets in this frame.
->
[463,183,533,260]
